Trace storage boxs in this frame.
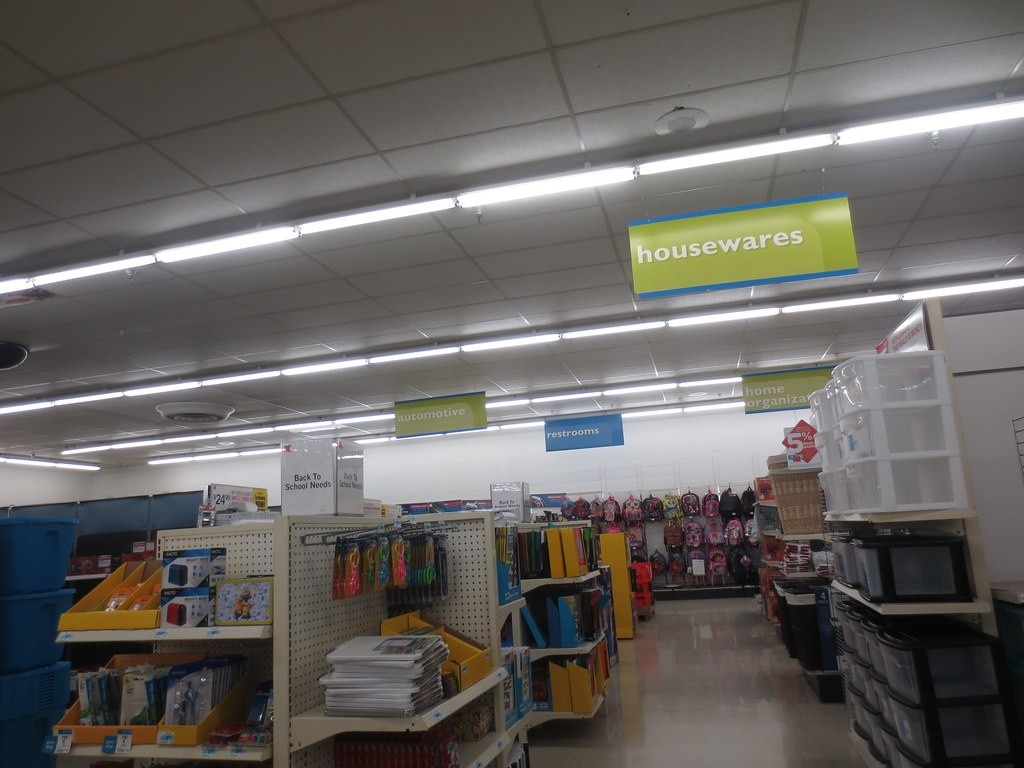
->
[757,350,1015,768]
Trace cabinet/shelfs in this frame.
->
[48,512,637,768]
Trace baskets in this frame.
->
[772,577,833,670]
[767,454,823,534]
